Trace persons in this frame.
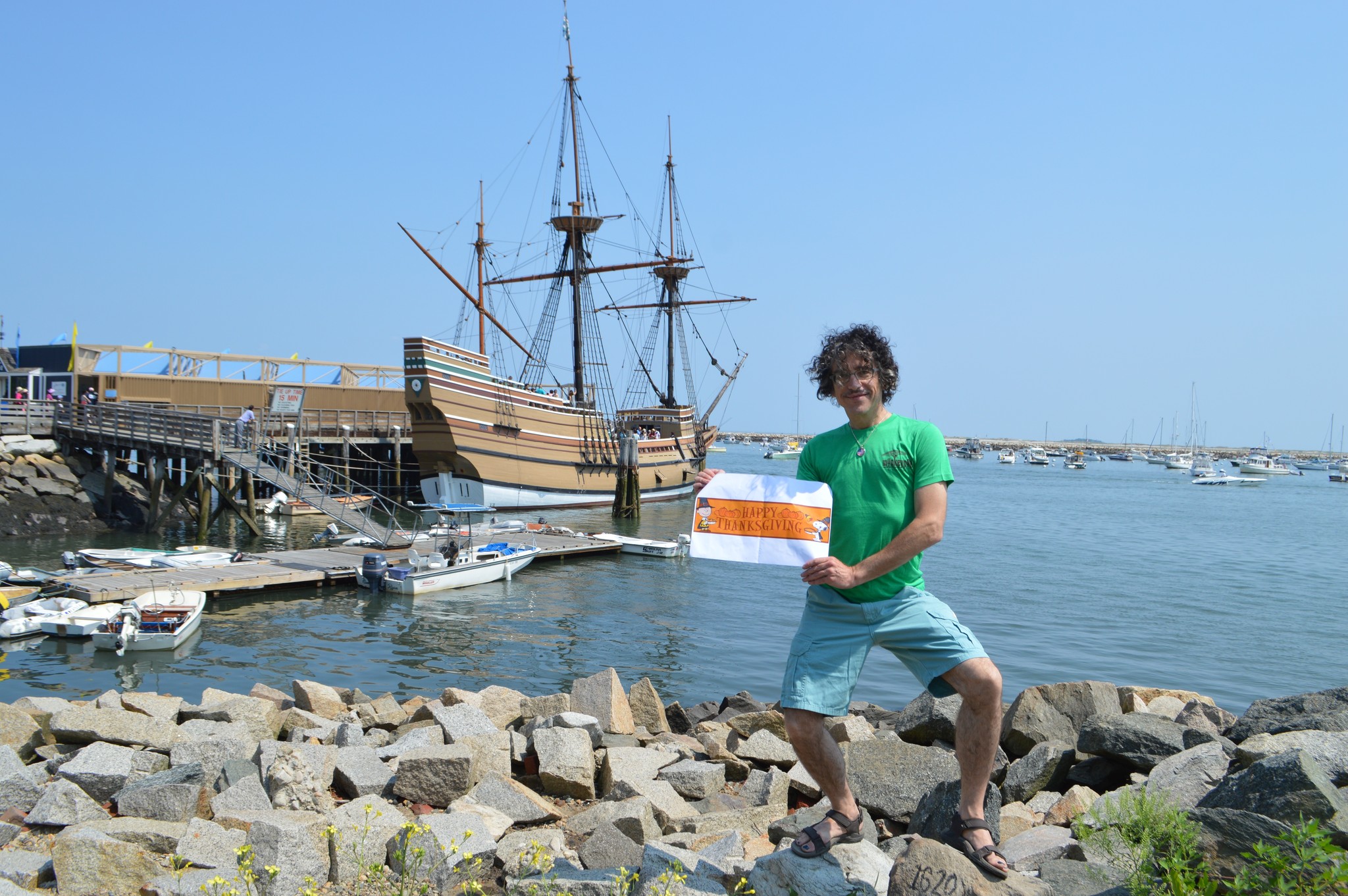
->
[234,405,256,448]
[3,377,101,427]
[689,323,1009,881]
[490,375,674,453]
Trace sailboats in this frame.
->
[404,0,758,513]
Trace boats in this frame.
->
[56,551,125,575]
[265,490,377,515]
[0,597,90,642]
[8,566,63,586]
[946,381,1348,485]
[431,522,490,531]
[0,587,41,609]
[112,547,193,557]
[78,548,166,571]
[312,523,437,545]
[490,515,525,530]
[91,579,207,656]
[525,518,607,540]
[41,603,123,639]
[592,533,691,558]
[354,501,541,597]
[0,561,13,585]
[706,443,727,453]
[407,501,520,536]
[176,546,237,556]
[150,552,243,568]
[716,433,808,452]
[764,447,801,459]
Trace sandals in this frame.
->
[791,798,865,858]
[940,811,1008,879]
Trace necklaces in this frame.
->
[848,411,890,457]
